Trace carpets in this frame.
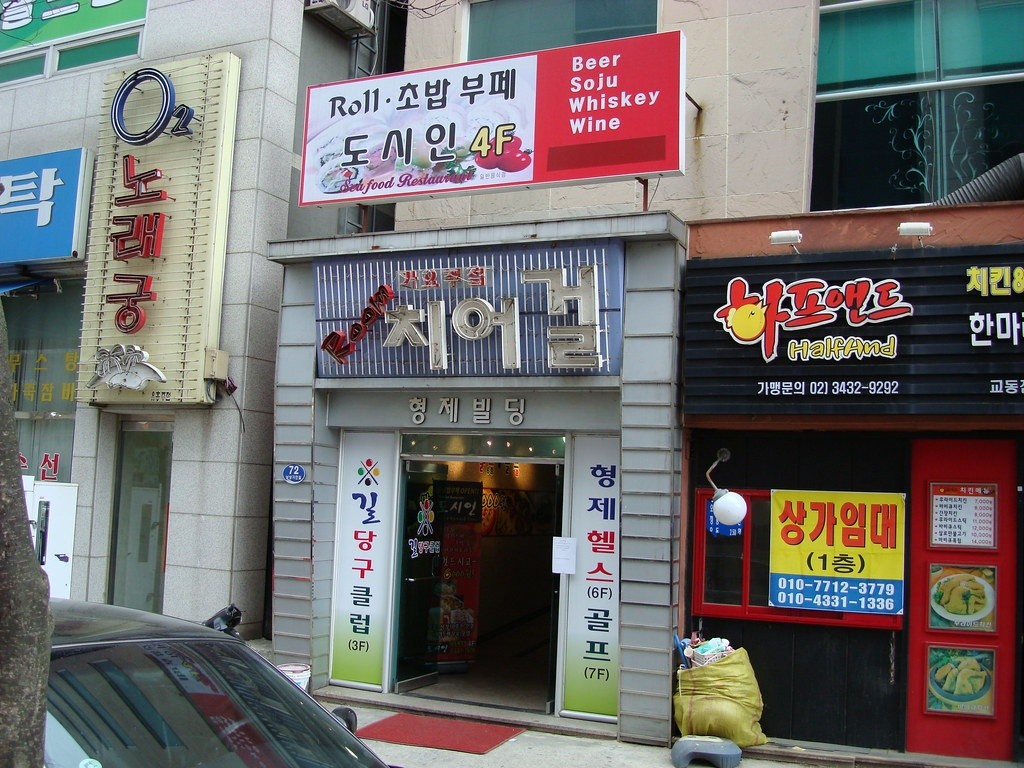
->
[354,712,526,755]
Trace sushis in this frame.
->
[313,106,468,193]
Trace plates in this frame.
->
[928,659,993,705]
[930,574,996,622]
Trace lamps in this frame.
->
[706,450,746,525]
[770,231,803,255]
[899,223,931,248]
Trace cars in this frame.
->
[34,589,397,768]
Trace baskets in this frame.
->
[691,646,735,668]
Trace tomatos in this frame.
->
[473,130,533,172]
